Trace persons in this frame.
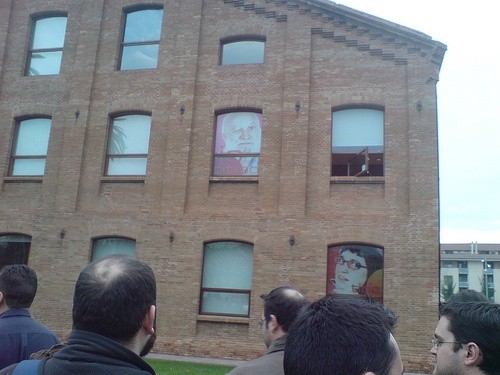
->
[0,264,62,370]
[225,286,309,375]
[331,244,384,302]
[220,111,262,175]
[0,256,157,375]
[431,289,500,375]
[283,295,407,375]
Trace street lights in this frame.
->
[481,259,491,299]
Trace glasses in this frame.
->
[257,319,268,329]
[432,334,482,356]
